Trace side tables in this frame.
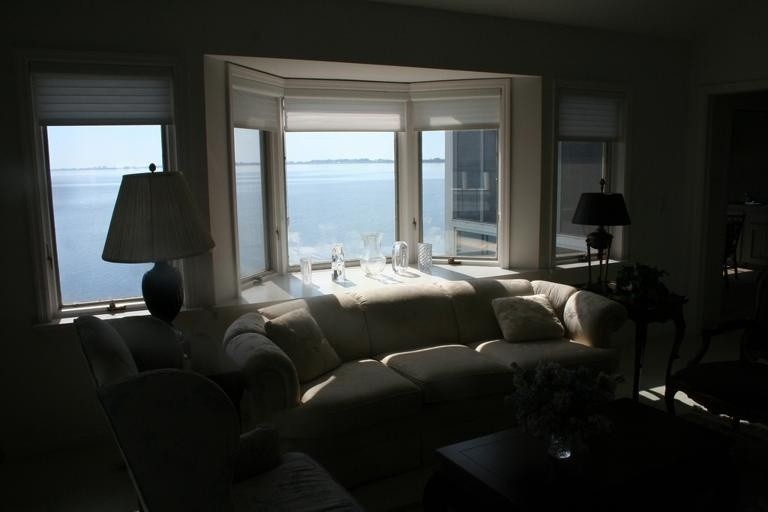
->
[177,334,251,434]
[572,282,689,402]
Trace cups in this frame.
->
[300,257,313,286]
[416,243,433,275]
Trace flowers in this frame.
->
[502,362,625,456]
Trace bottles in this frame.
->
[329,243,347,284]
[392,240,410,274]
[359,230,387,277]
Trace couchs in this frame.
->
[223,278,627,489]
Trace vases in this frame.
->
[300,232,432,285]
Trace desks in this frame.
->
[423,398,741,512]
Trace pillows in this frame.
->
[491,294,565,342]
[264,308,342,383]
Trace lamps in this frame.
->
[102,164,215,328]
[572,179,631,297]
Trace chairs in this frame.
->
[724,215,744,287]
[665,271,768,433]
[75,315,364,512]
